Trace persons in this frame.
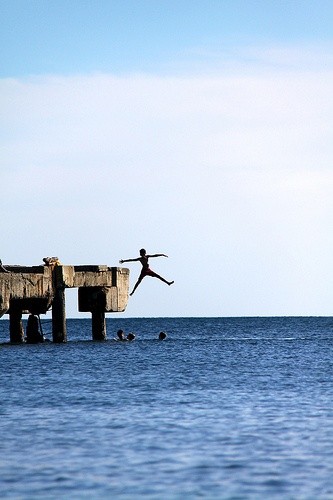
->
[119,248,175,296]
[126,333,136,341]
[116,329,126,341]
[158,332,166,340]
